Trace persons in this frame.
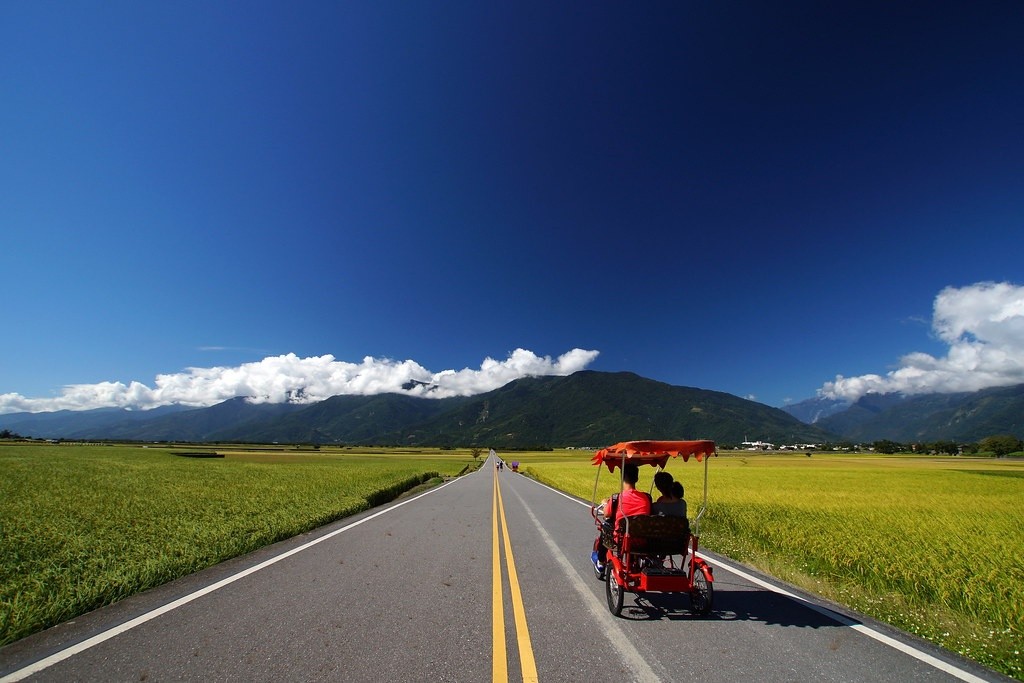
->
[496,461,503,470]
[651,472,687,560]
[591,463,653,573]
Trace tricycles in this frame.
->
[591,440,719,618]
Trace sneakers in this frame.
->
[591,552,605,573]
